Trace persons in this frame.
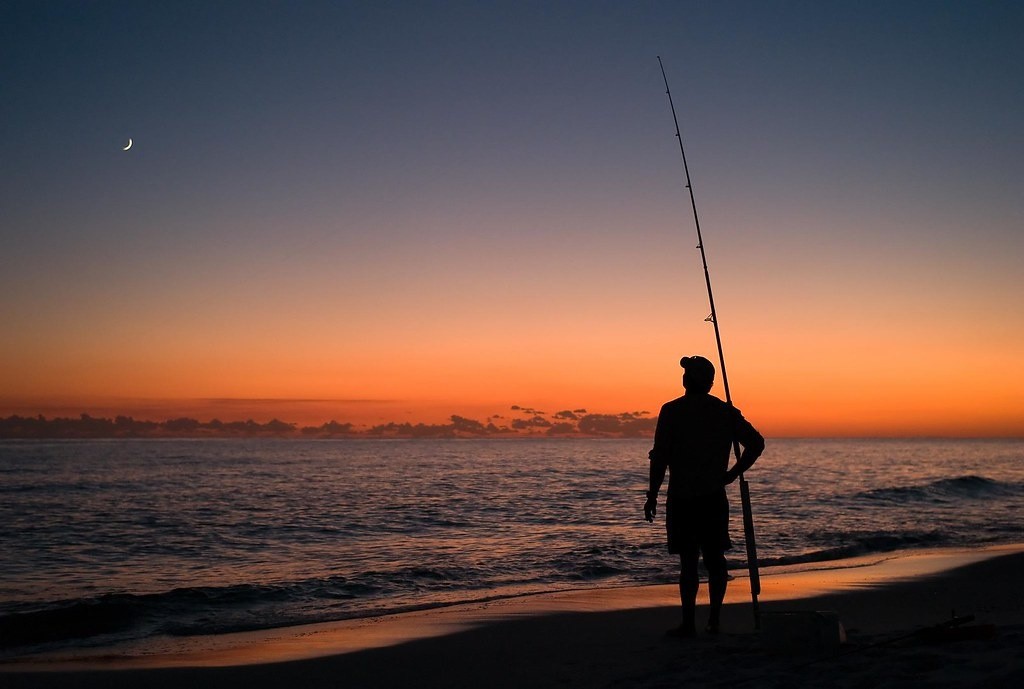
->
[644,356,765,639]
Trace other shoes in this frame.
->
[665,624,698,639]
[706,618,720,634]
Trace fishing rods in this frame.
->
[656,54,764,636]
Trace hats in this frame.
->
[680,356,715,381]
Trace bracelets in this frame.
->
[647,492,658,499]
